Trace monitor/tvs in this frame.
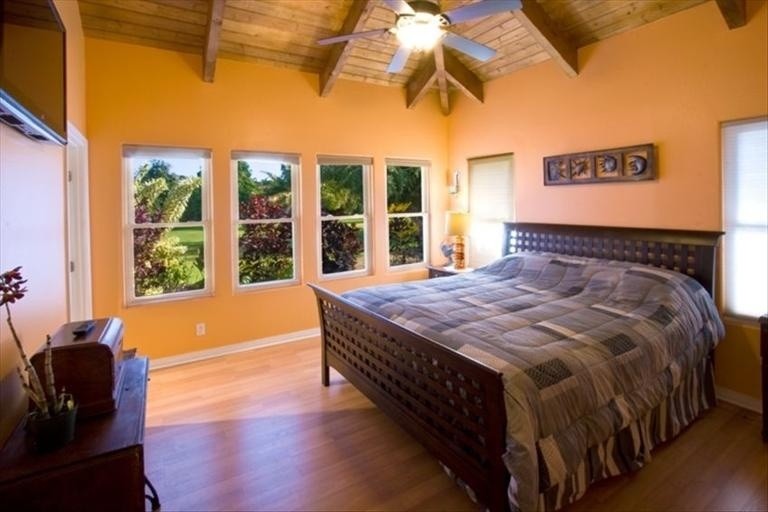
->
[0,0,67,146]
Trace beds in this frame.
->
[305,220,727,511]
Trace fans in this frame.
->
[317,0,523,75]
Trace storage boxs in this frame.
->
[29,316,127,417]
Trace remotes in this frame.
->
[73,320,97,335]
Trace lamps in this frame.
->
[396,20,441,52]
[444,211,475,270]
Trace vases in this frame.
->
[29,404,79,452]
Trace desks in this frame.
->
[0,356,161,511]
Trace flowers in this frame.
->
[0,266,68,418]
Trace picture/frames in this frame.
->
[543,142,655,186]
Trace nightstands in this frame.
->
[426,262,475,279]
